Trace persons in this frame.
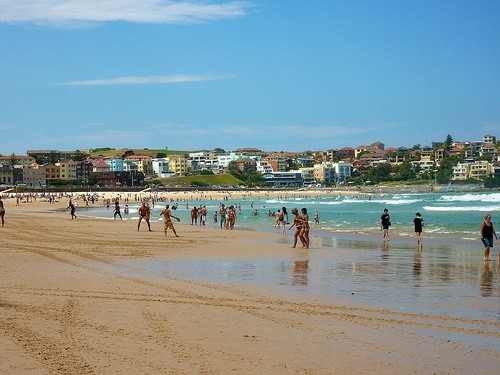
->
[480,213,498,261]
[381,208,391,241]
[412,212,424,248]
[0,192,372,248]
